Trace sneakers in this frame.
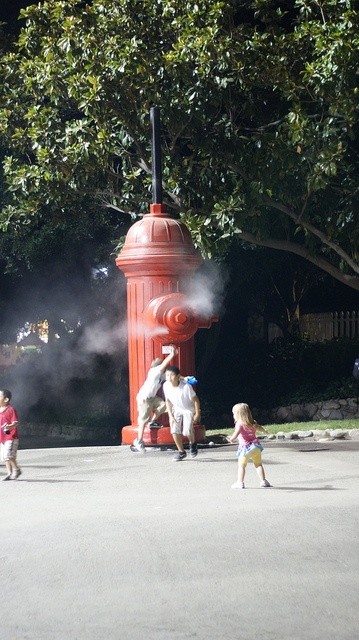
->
[260,480,270,487]
[173,450,187,460]
[231,481,244,489]
[133,438,146,451]
[9,468,21,481]
[3,473,11,480]
[148,419,164,428]
[188,441,198,458]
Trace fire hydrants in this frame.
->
[115,108,219,445]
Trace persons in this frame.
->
[224,403,273,488]
[0,389,23,481]
[132,344,180,453]
[161,365,202,461]
